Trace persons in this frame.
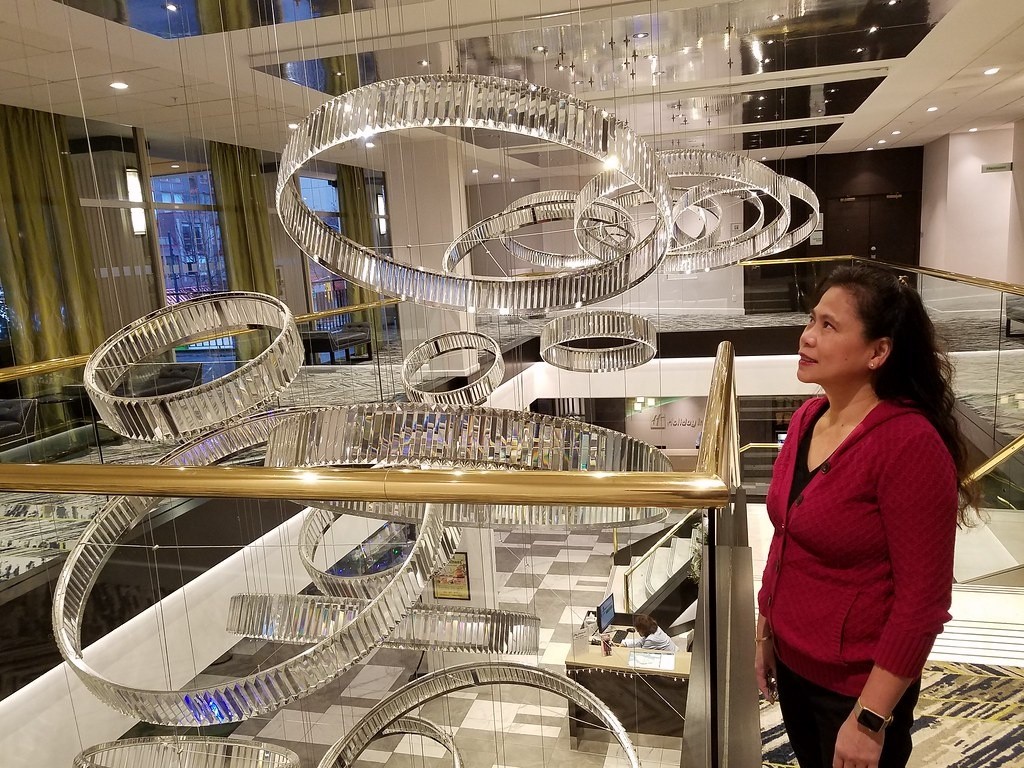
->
[755,265,981,768]
[614,614,679,652]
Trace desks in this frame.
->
[36,393,88,446]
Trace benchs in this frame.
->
[63,363,203,445]
[0,399,37,447]
[299,322,373,366]
[1006,295,1024,336]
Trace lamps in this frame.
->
[377,193,387,235]
[126,166,148,236]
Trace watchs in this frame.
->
[854,697,894,733]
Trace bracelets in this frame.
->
[754,635,770,641]
